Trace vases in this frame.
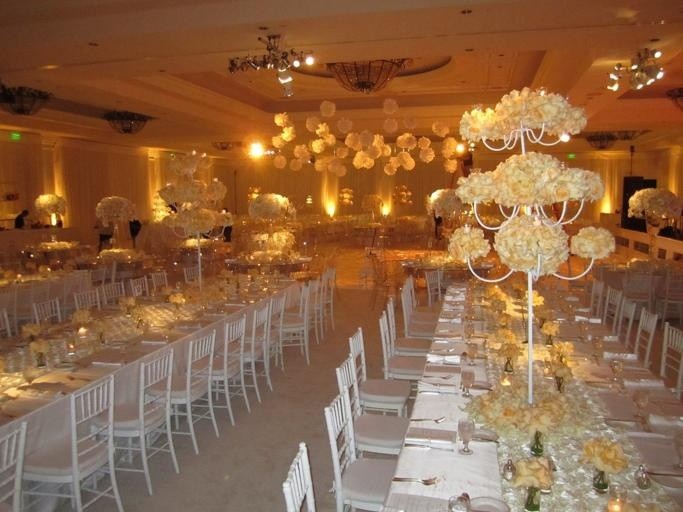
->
[591,472,611,493]
[97,333,107,345]
[528,433,545,456]
[553,375,565,393]
[525,487,541,511]
[36,354,46,368]
[136,319,146,329]
[502,356,514,372]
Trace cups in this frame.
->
[447,494,470,512]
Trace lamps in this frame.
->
[605,48,665,94]
[0,84,50,116]
[586,133,618,151]
[326,57,414,95]
[226,33,314,100]
[666,86,683,116]
[213,141,234,151]
[102,110,148,135]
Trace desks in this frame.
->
[0,268,75,314]
[0,264,326,511]
[384,279,682,512]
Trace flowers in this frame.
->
[293,144,308,162]
[380,144,392,157]
[280,126,296,142]
[168,292,185,311]
[542,321,560,340]
[272,155,286,169]
[420,149,434,163]
[94,320,113,345]
[323,134,336,147]
[264,230,300,261]
[23,324,40,342]
[367,134,384,158]
[572,223,616,260]
[443,159,457,174]
[169,149,212,176]
[345,134,359,150]
[381,98,400,115]
[339,188,356,208]
[333,143,348,159]
[247,192,294,221]
[134,307,150,329]
[362,194,382,211]
[318,100,335,119]
[430,119,449,138]
[396,134,417,151]
[159,210,233,234]
[358,130,372,148]
[391,182,415,210]
[470,280,557,322]
[626,184,682,227]
[120,297,134,317]
[440,136,456,159]
[336,165,347,178]
[402,114,417,130]
[498,343,522,372]
[509,453,554,493]
[158,178,227,206]
[313,158,325,173]
[457,87,587,141]
[417,137,430,150]
[448,224,490,264]
[273,111,288,128]
[73,310,89,324]
[310,139,325,154]
[469,376,581,444]
[425,189,465,223]
[94,195,138,226]
[382,118,398,133]
[328,160,341,174]
[337,118,353,133]
[315,124,329,137]
[493,215,571,277]
[288,158,303,172]
[455,152,605,207]
[578,437,628,473]
[389,156,400,168]
[397,151,415,171]
[353,151,374,170]
[484,328,514,352]
[304,115,319,133]
[34,192,67,216]
[270,134,285,149]
[29,339,50,369]
[382,164,396,176]
[211,268,269,297]
[549,342,576,371]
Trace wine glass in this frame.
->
[463,278,474,340]
[465,342,477,366]
[461,368,474,397]
[671,431,683,475]
[547,284,588,341]
[590,335,604,362]
[631,388,648,420]
[457,418,474,456]
[610,357,625,390]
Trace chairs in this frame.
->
[188,312,252,427]
[100,280,125,304]
[1,285,20,336]
[636,278,660,306]
[587,278,604,316]
[385,295,431,356]
[622,278,636,295]
[660,320,682,397]
[261,294,286,376]
[20,280,50,322]
[333,352,407,452]
[603,283,622,333]
[33,297,63,325]
[400,286,436,337]
[584,263,682,278]
[634,306,659,366]
[403,273,439,322]
[323,385,396,512]
[276,281,310,368]
[281,440,317,512]
[90,348,178,495]
[184,264,201,284]
[617,295,636,346]
[72,287,100,314]
[346,327,410,417]
[21,374,124,512]
[315,265,336,335]
[149,270,169,289]
[215,297,273,403]
[64,267,106,287]
[0,309,15,337]
[300,277,325,343]
[423,269,443,307]
[129,274,148,296]
[0,419,29,512]
[142,329,220,454]
[660,279,683,321]
[377,309,424,398]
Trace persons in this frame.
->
[14,209,28,229]
[221,207,233,242]
